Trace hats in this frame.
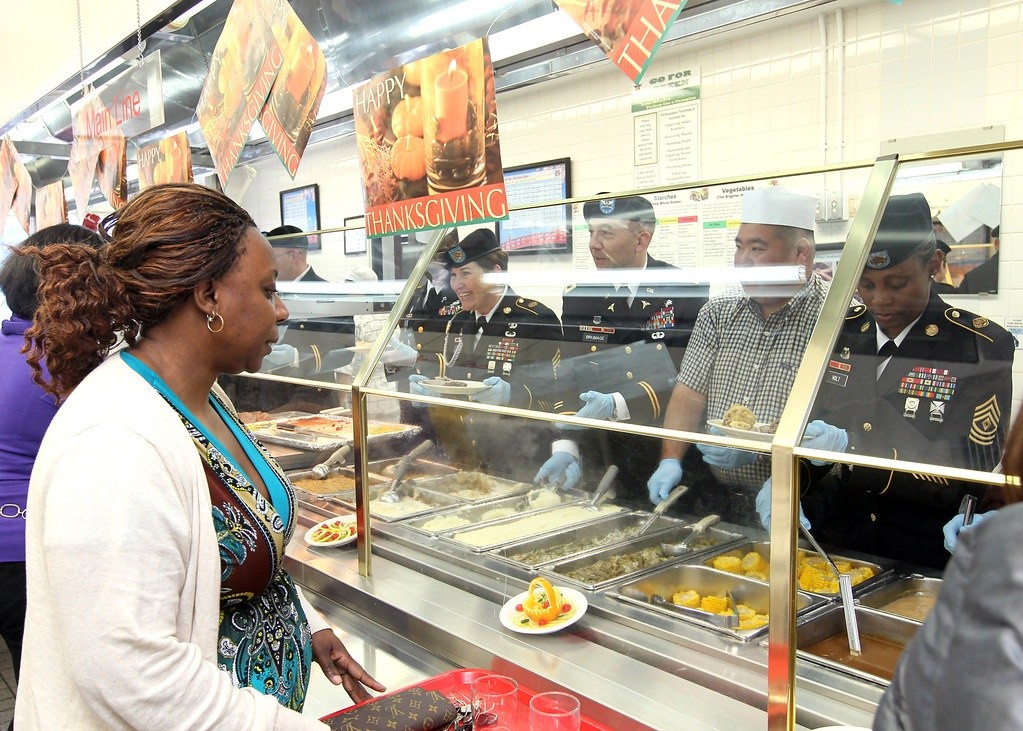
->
[444,229,501,269]
[990,226,1000,238]
[863,191,935,270]
[416,230,458,260]
[584,189,657,223]
[264,226,310,248]
[734,186,818,234]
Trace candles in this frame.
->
[286,42,314,105]
[170,144,181,179]
[433,58,468,143]
[232,16,252,63]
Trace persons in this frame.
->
[334,263,419,423]
[380,227,463,460]
[534,191,709,517]
[872,502,1023,731]
[234,225,356,414]
[0,224,106,731]
[647,190,860,530]
[13,183,387,731]
[756,193,1015,571]
[958,225,999,295]
[931,240,958,294]
[408,228,563,486]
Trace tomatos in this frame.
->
[322,521,355,539]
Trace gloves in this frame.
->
[553,392,617,432]
[942,508,999,554]
[648,456,682,505]
[533,448,582,491]
[800,421,848,466]
[381,336,417,370]
[257,343,296,373]
[753,477,810,534]
[692,428,759,469]
[408,374,442,409]
[467,376,514,408]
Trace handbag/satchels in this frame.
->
[321,684,458,731]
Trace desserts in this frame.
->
[722,404,755,431]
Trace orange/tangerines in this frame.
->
[521,578,563,622]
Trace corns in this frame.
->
[671,552,874,632]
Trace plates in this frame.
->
[345,344,395,353]
[498,585,588,635]
[707,418,819,445]
[303,513,371,547]
[417,380,494,394]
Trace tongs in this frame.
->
[838,574,862,658]
[276,422,347,441]
[650,589,739,628]
[798,520,846,592]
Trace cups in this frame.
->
[471,674,581,731]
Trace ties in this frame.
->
[471,315,489,350]
[421,286,438,315]
[875,340,898,368]
[616,286,632,311]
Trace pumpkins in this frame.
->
[153,134,179,184]
[388,59,431,183]
[218,56,242,119]
